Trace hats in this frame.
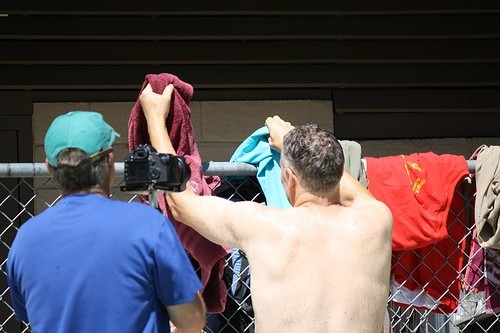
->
[44,111,120,170]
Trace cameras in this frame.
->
[121,144,191,192]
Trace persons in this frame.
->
[7,111,206,333]
[139,83,394,333]
